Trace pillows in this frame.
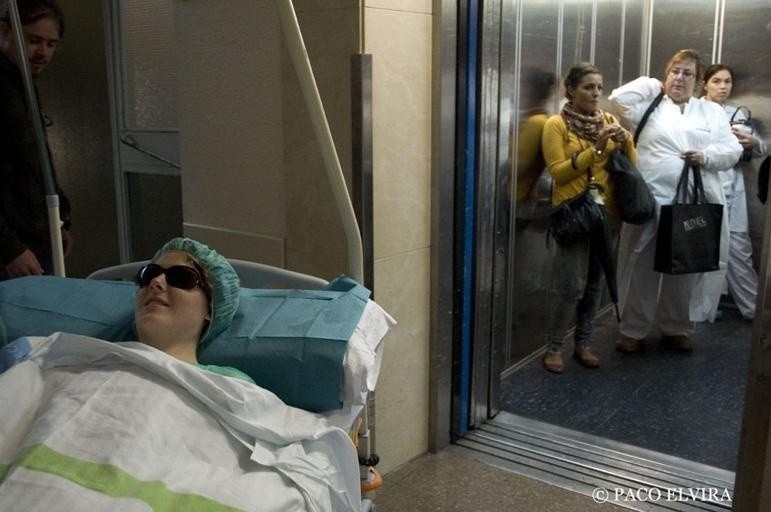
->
[0,271,394,408]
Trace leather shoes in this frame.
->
[542,350,564,374]
[574,346,601,367]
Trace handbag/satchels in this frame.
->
[542,182,612,248]
[603,148,658,226]
[653,203,725,276]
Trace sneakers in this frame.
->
[614,332,639,353]
[672,334,698,352]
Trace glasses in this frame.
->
[134,262,212,301]
[668,68,700,81]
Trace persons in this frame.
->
[608,49,746,357]
[0,0,74,281]
[541,60,637,374]
[132,237,257,385]
[698,64,767,324]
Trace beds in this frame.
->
[1,254,384,509]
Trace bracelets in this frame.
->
[597,150,606,158]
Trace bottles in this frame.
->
[590,176,599,197]
